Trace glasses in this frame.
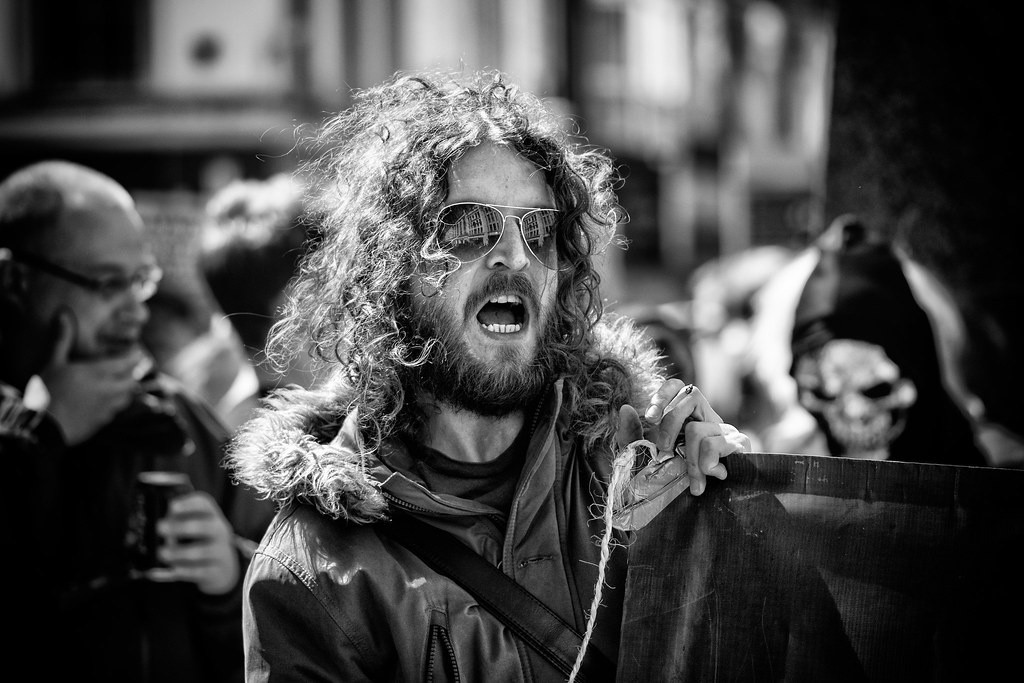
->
[423,201,582,272]
[7,246,162,303]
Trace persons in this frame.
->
[236,68,871,683]
[0,159,1024,683]
